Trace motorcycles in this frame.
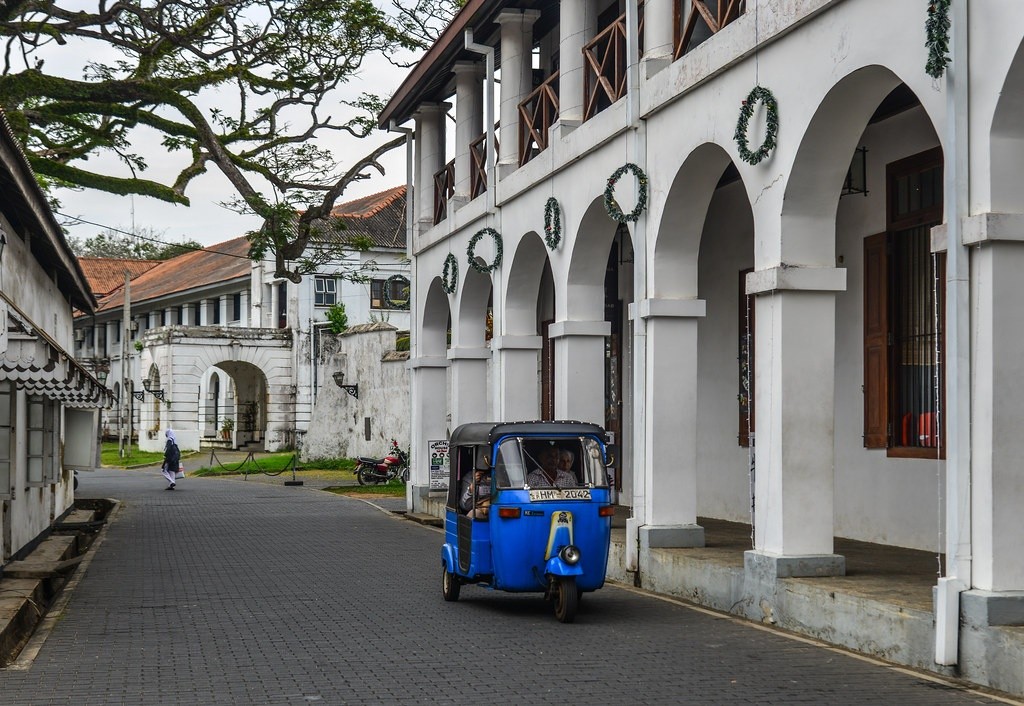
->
[440,419,616,623]
[353,438,411,487]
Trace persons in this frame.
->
[518,444,575,494]
[549,449,579,489]
[461,452,503,522]
[159,429,180,491]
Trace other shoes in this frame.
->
[165,484,176,490]
